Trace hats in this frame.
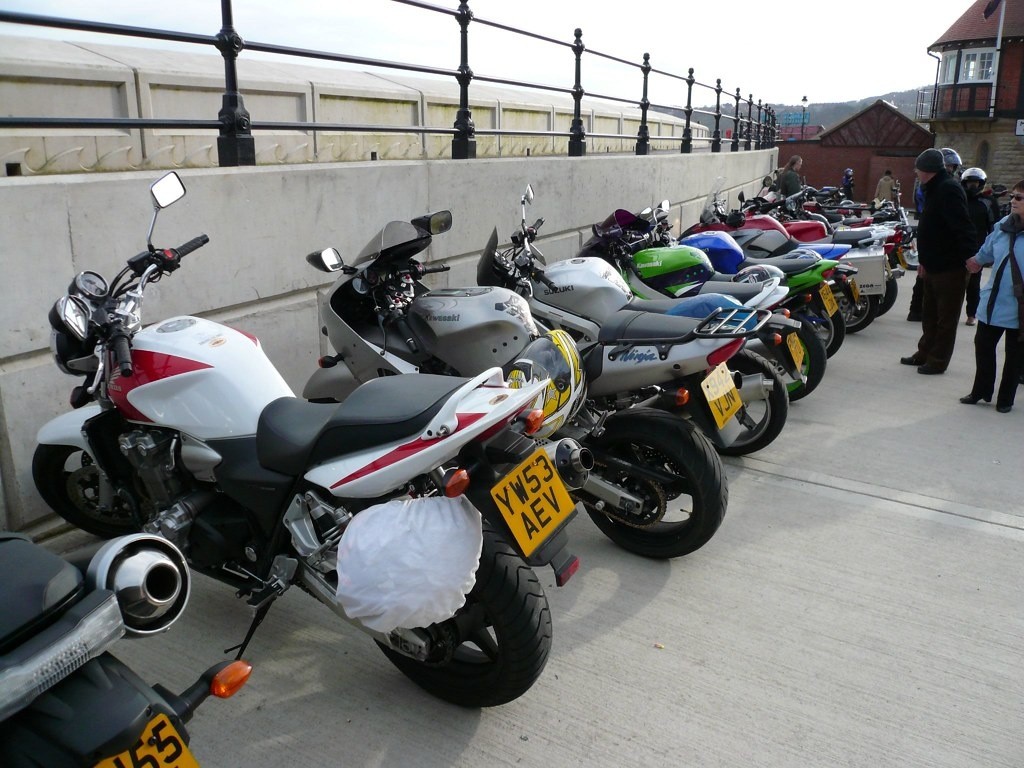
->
[916,148,946,172]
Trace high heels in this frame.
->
[997,407,1011,413]
[960,392,991,404]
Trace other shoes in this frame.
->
[966,317,975,326]
[901,356,925,365]
[907,311,922,321]
[918,363,944,374]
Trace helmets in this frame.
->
[506,330,587,441]
[962,168,987,181]
[940,148,962,165]
[845,169,853,175]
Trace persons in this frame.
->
[781,155,803,198]
[960,180,1024,413]
[900,147,1001,374]
[841,168,855,200]
[874,170,895,201]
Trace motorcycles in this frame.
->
[477,184,806,457]
[29,171,579,708]
[301,210,730,559]
[675,166,919,334]
[0,522,251,768]
[576,201,845,401]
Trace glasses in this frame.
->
[1010,194,1022,201]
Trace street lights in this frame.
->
[801,95,808,140]
